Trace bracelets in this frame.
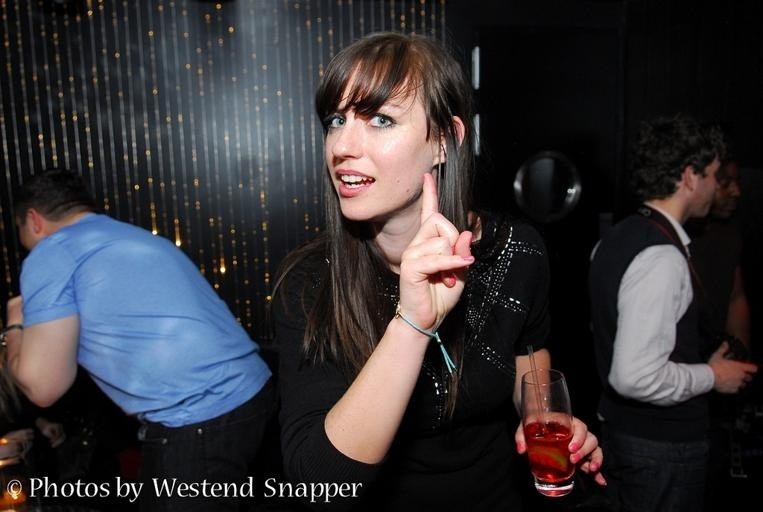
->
[4,324,22,331]
[394,302,438,340]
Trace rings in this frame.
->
[16,443,23,452]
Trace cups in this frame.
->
[522,369,576,499]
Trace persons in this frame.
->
[257,30,609,512]
[0,317,67,471]
[582,109,758,511]
[683,143,753,364]
[2,167,280,512]
